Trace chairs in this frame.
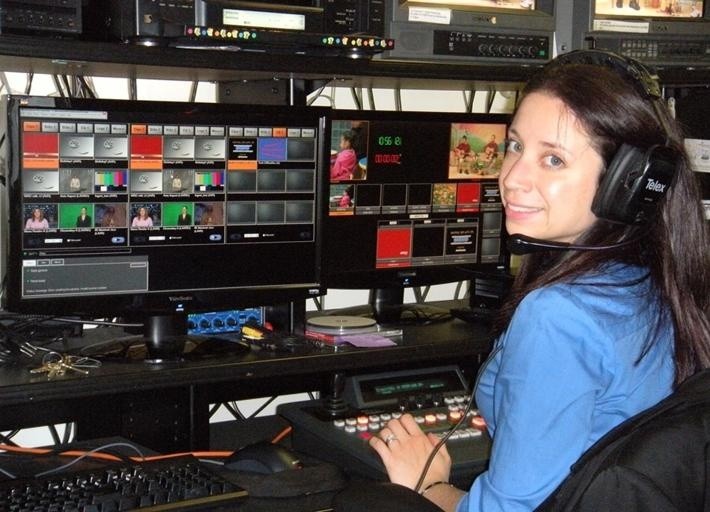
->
[332,368,710,512]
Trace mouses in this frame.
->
[224,439,304,473]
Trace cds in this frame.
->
[307,314,380,336]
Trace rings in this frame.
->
[384,435,397,444]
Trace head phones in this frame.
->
[557,49,677,232]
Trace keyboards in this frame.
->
[0,453,250,512]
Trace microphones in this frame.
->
[504,231,651,256]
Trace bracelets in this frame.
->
[419,479,453,496]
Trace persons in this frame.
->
[26,207,49,230]
[366,51,710,512]
[101,206,120,227]
[132,206,153,227]
[340,188,352,207]
[328,129,360,180]
[456,134,469,174]
[178,206,191,224]
[76,207,91,227]
[349,120,367,160]
[485,134,499,161]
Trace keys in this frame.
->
[29,351,102,378]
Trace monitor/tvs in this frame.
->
[4,93,334,365]
[593,0,709,23]
[403,0,538,12]
[324,106,516,332]
